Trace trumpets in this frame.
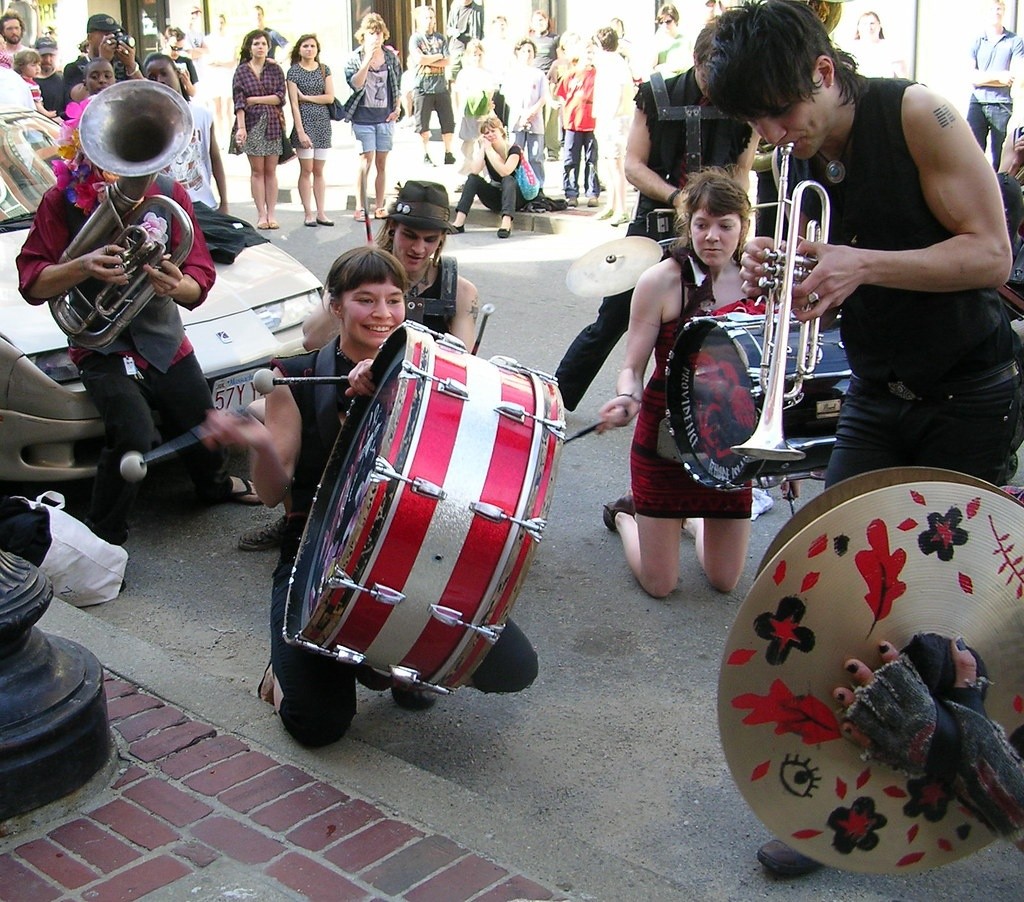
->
[728,142,833,462]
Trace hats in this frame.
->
[87,14,124,30]
[382,181,457,234]
[35,37,57,56]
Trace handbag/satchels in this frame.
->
[7,491,129,608]
[319,63,346,121]
[509,144,540,200]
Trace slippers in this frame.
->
[228,475,266,504]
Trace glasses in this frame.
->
[166,39,183,51]
[659,19,672,25]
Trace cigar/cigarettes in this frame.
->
[235,140,246,144]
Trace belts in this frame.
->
[849,361,1019,400]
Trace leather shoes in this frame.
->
[603,495,635,531]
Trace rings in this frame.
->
[808,292,819,304]
[104,245,109,254]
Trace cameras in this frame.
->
[108,29,135,48]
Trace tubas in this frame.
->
[50,76,198,351]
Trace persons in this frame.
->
[954,0,1024,173]
[841,11,907,80]
[238,180,480,552]
[554,20,761,412]
[0,7,229,217]
[405,0,692,239]
[833,636,1024,852]
[595,167,781,599]
[233,6,402,230]
[451,114,527,239]
[693,2,1024,875]
[199,244,541,753]
[15,97,262,594]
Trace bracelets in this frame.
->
[79,254,90,279]
[126,62,139,75]
[238,127,245,129]
[617,393,641,412]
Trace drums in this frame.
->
[663,311,853,496]
[281,319,569,698]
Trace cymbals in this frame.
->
[564,235,664,300]
[715,461,1023,875]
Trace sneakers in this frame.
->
[355,208,366,221]
[373,207,389,218]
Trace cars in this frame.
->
[0,68,330,507]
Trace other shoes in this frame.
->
[305,221,317,226]
[568,197,579,206]
[588,197,599,207]
[258,220,269,229]
[316,217,334,226]
[498,228,510,238]
[445,152,456,164]
[267,219,279,229]
[451,225,464,233]
[238,516,288,550]
